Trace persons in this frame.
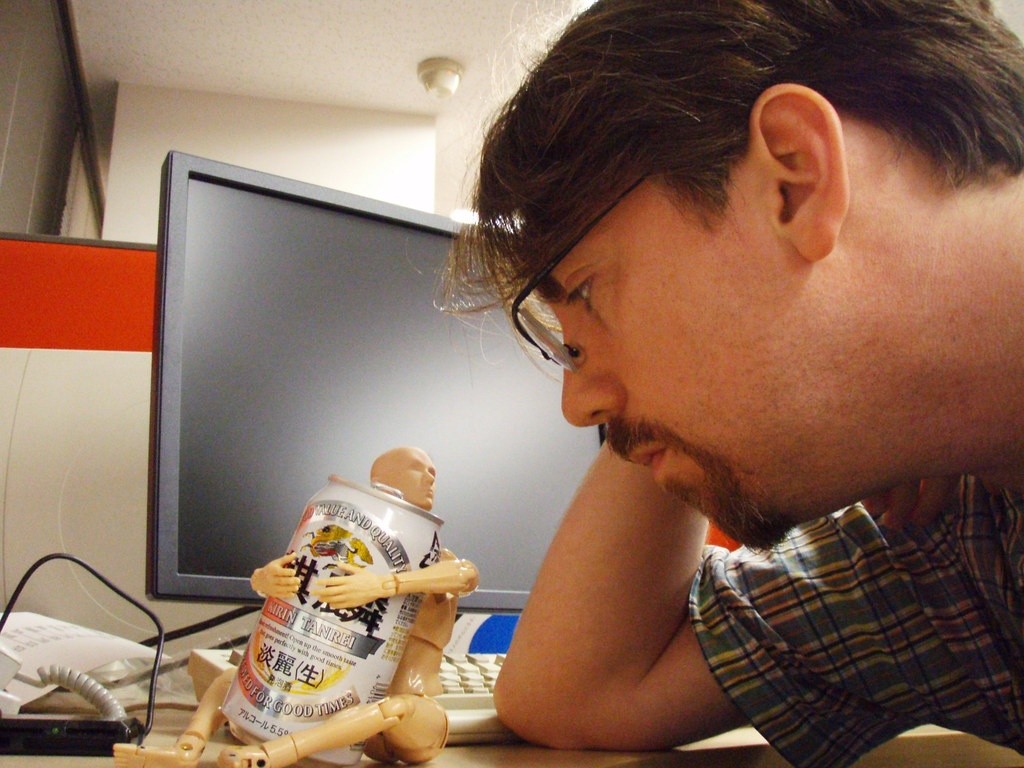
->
[437,0,1024,768]
[113,447,479,768]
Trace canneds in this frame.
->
[222,476,445,768]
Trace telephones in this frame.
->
[0,646,24,715]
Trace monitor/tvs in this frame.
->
[144,150,610,611]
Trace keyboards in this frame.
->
[189,648,523,746]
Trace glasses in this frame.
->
[510,168,653,375]
